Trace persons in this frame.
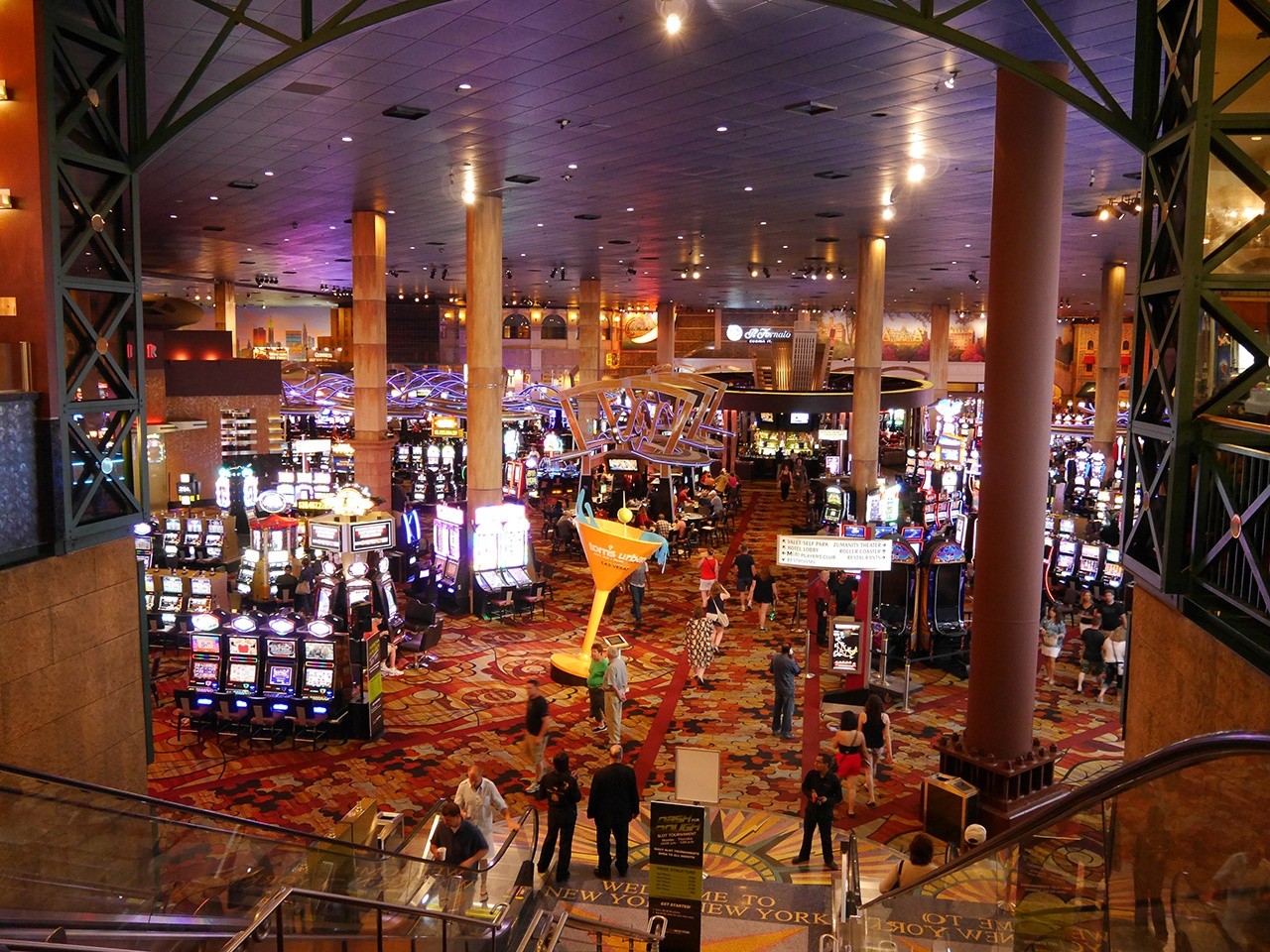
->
[537,752,581,882]
[602,646,627,749]
[429,803,489,930]
[587,745,640,879]
[276,417,1128,703]
[770,643,800,739]
[454,765,520,902]
[1131,802,1270,952]
[684,605,713,687]
[586,643,609,733]
[857,694,892,805]
[525,680,548,793]
[876,823,1007,952]
[830,710,867,817]
[791,753,843,867]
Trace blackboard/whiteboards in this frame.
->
[674,746,720,804]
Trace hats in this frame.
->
[964,824,987,844]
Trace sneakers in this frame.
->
[592,726,607,733]
[526,783,540,792]
[381,665,389,675]
[389,668,404,676]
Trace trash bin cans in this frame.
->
[919,772,980,848]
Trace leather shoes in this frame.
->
[782,732,793,738]
[824,861,838,870]
[773,728,781,735]
[791,856,809,863]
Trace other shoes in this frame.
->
[866,802,876,807]
[848,812,855,817]
[594,867,611,880]
[696,677,704,685]
[747,605,752,610]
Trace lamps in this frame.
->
[505,267,565,309]
[627,268,638,276]
[772,305,856,316]
[1058,300,1071,308]
[430,268,437,279]
[945,70,960,90]
[746,267,847,279]
[968,271,981,284]
[247,276,279,299]
[389,270,399,278]
[312,284,353,300]
[1097,193,1139,221]
[441,268,449,281]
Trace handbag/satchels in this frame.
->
[831,764,837,772]
[769,607,776,621]
[882,860,904,907]
[1043,636,1058,646]
[1118,664,1124,675]
[718,613,729,627]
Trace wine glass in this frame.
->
[548,517,665,679]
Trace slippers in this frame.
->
[480,892,488,902]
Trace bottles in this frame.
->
[812,789,817,802]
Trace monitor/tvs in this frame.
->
[239,570,286,585]
[1044,538,1124,589]
[144,575,212,614]
[316,582,397,622]
[190,634,333,690]
[136,536,152,572]
[506,464,521,482]
[822,457,967,624]
[163,518,224,547]
[434,526,533,588]
[276,472,331,507]
[608,459,640,472]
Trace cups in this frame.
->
[437,847,446,861]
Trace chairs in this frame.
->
[147,613,330,753]
[650,484,743,565]
[399,598,443,670]
[538,474,564,504]
[488,507,585,623]
[1060,575,1106,626]
